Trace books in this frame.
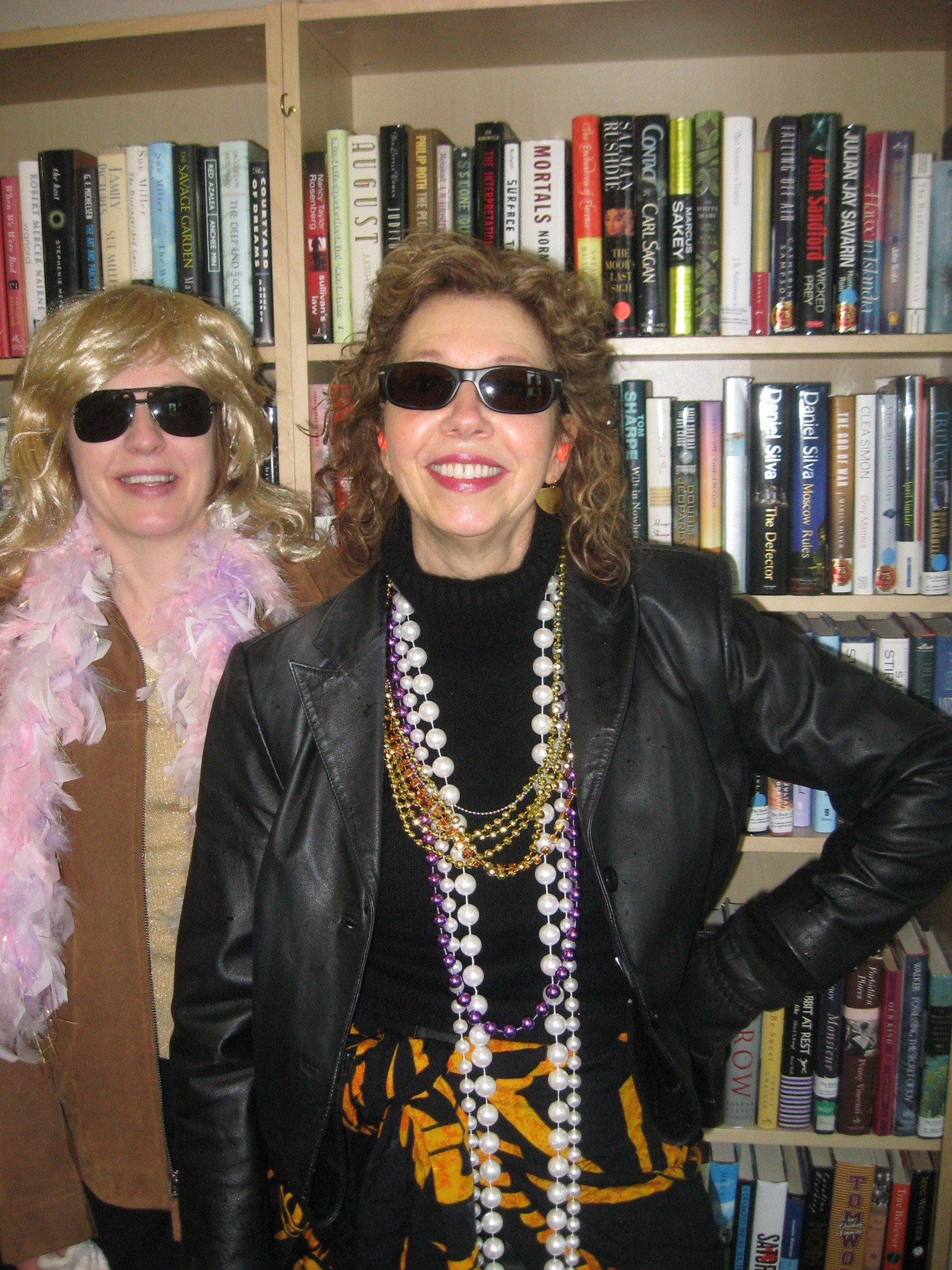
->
[698,615,950,1270]
[0,110,951,599]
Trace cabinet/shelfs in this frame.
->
[5,2,952,1270]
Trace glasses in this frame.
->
[377,360,564,415]
[70,385,220,444]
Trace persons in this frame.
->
[163,235,950,1270]
[0,278,335,1268]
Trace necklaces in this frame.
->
[367,537,589,1269]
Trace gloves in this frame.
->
[678,889,814,1127]
